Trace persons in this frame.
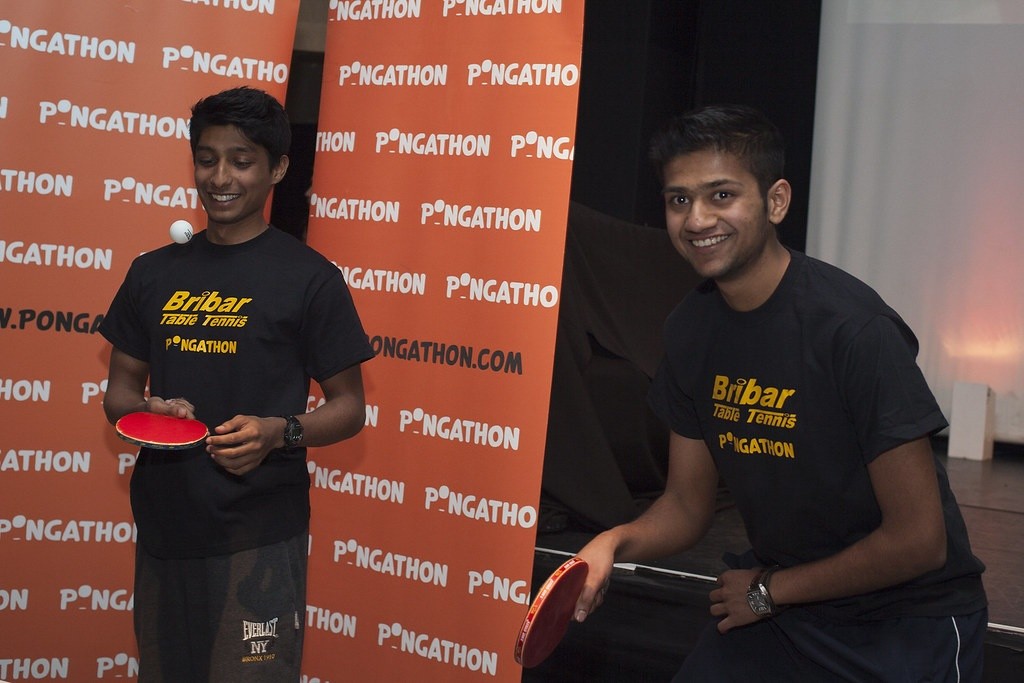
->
[93,83,380,683]
[564,98,1002,682]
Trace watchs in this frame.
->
[746,559,785,620]
[280,411,305,452]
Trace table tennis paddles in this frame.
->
[115,396,210,452]
[514,558,613,669]
